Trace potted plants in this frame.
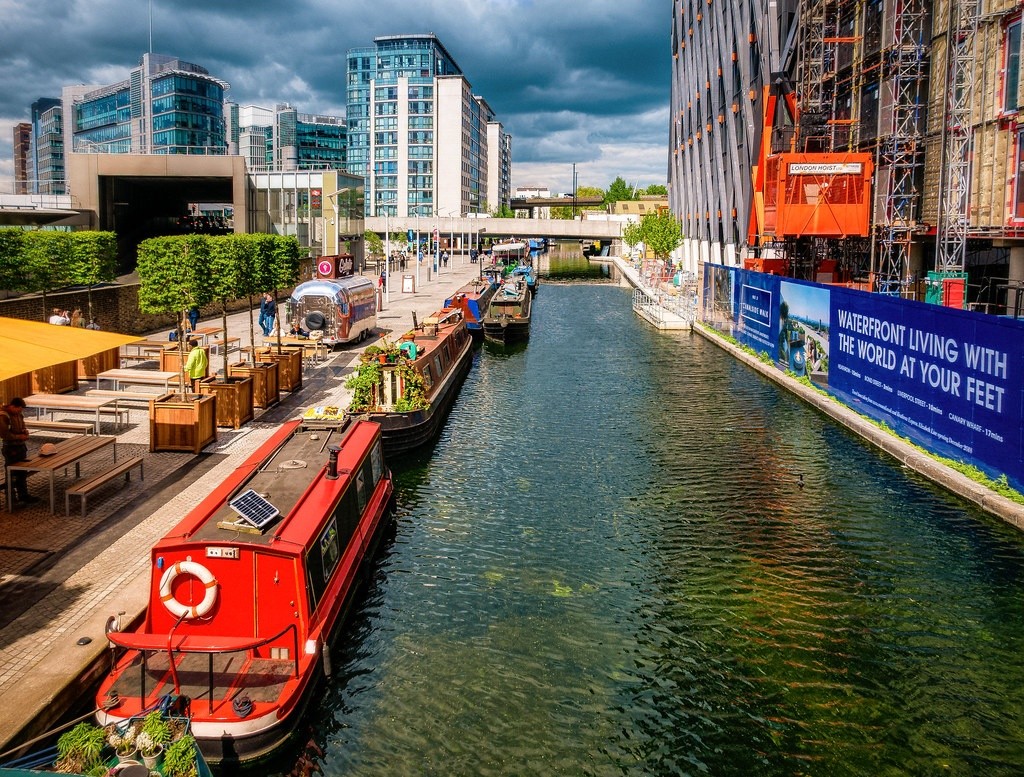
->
[138,233,319,454]
[0,228,124,404]
[56,710,197,777]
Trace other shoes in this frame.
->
[5,498,26,510]
[18,494,41,505]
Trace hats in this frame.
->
[189,339,198,347]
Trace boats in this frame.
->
[527,239,545,249]
[95,407,396,768]
[0,692,217,777]
[346,308,473,457]
[443,242,537,346]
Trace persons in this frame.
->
[470,248,479,264]
[381,269,386,293]
[49,308,100,330]
[290,324,305,335]
[187,297,200,331]
[419,244,426,262]
[439,249,448,267]
[269,321,286,337]
[258,291,276,336]
[0,398,41,509]
[183,340,208,393]
[382,254,405,272]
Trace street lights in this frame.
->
[375,197,396,303]
[325,188,350,254]
[411,203,425,290]
[467,213,477,257]
[459,211,468,265]
[432,206,446,278]
[448,209,456,271]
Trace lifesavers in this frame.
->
[157,561,218,619]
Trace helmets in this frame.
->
[37,443,57,456]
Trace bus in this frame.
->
[283,275,378,345]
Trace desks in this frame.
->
[126,341,178,370]
[241,346,306,373]
[97,367,179,406]
[187,326,224,355]
[7,434,117,517]
[16,395,118,436]
[263,337,323,368]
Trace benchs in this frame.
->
[16,336,334,521]
